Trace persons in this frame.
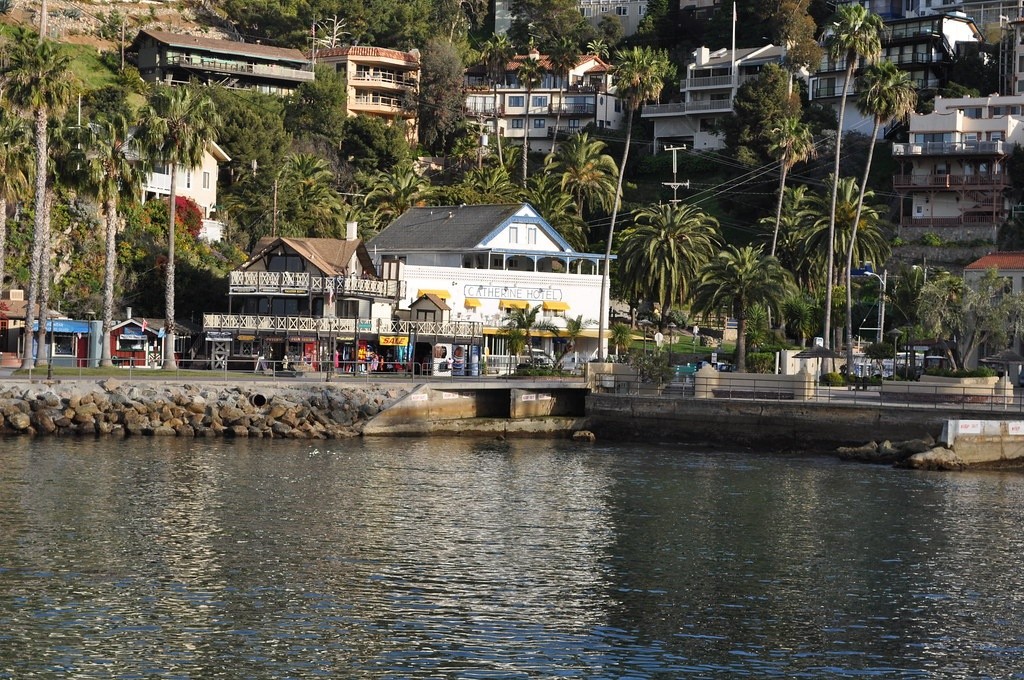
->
[692,323,700,344]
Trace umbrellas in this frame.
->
[792,344,842,371]
[978,349,1024,410]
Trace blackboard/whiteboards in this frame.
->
[260,359,267,371]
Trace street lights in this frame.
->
[888,328,903,380]
[47,309,59,380]
[903,322,913,381]
[771,326,778,346]
[84,308,96,370]
[638,318,653,382]
[324,313,337,382]
[666,323,677,368]
[864,268,888,343]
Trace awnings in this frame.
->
[543,301,570,311]
[32,319,93,334]
[120,334,147,341]
[499,299,531,309]
[464,298,481,308]
[416,289,451,299]
[483,328,653,340]
